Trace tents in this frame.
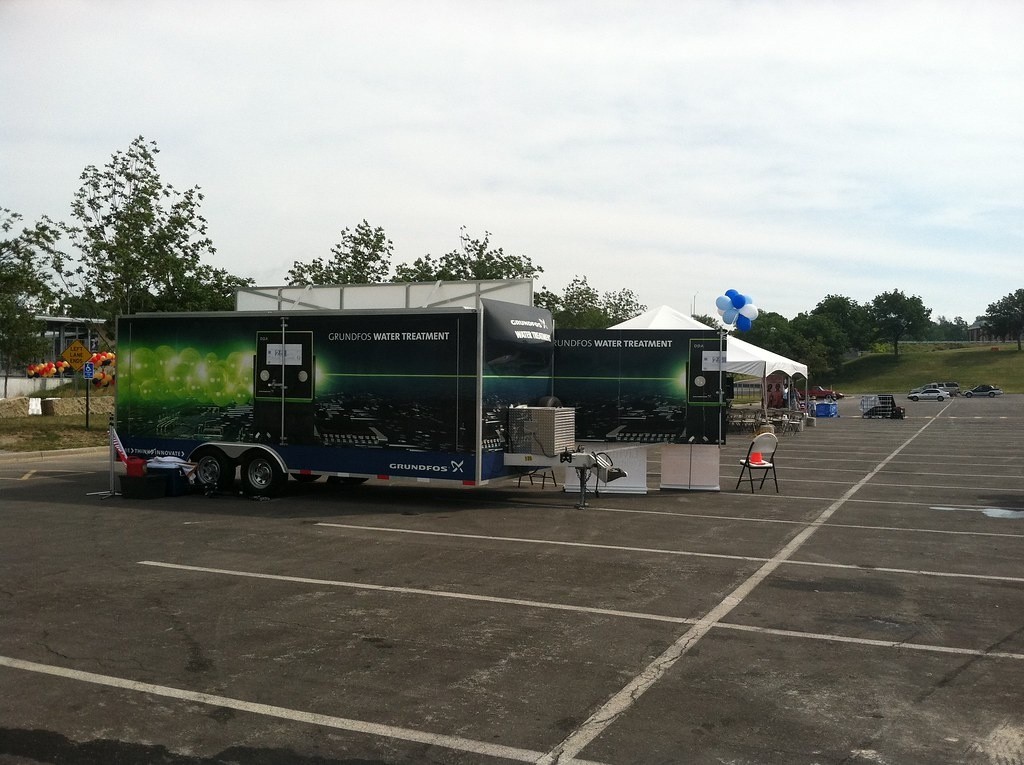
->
[606,304,808,421]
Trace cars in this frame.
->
[907,389,950,401]
[962,384,1003,397]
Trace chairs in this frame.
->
[726,408,805,436]
[736,433,778,494]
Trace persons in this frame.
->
[783,383,788,408]
[773,383,782,404]
[767,384,773,404]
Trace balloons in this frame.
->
[27,361,70,377]
[88,351,115,387]
[715,288,758,332]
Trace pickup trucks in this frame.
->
[798,385,836,399]
[910,383,958,396]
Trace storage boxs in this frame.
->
[118,473,168,499]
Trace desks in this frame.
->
[815,403,837,418]
